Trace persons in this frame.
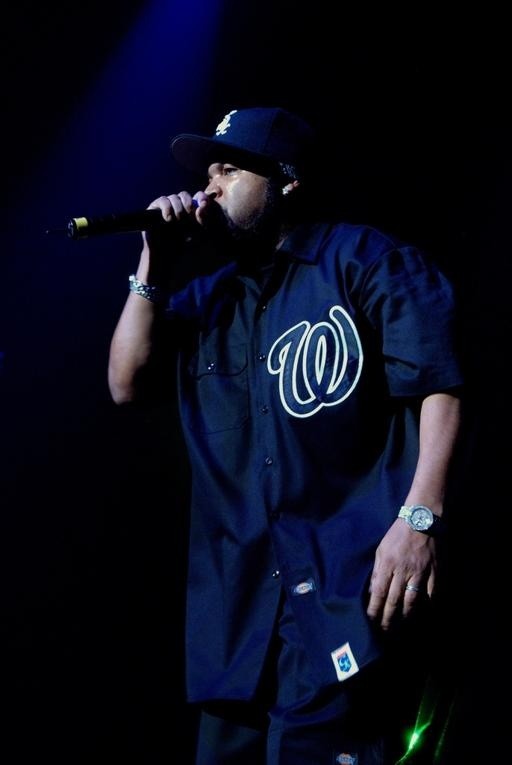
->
[105,104,465,759]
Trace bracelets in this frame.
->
[128,274,170,308]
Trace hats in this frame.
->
[170,106,316,175]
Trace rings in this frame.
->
[406,585,419,592]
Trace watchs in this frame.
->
[396,506,443,539]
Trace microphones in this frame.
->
[65,200,222,240]
